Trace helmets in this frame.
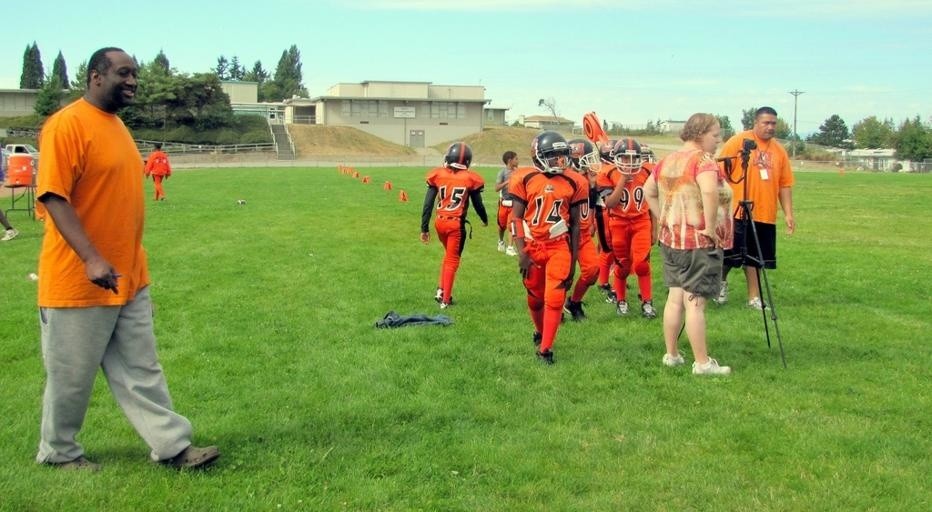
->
[444,143,472,170]
[531,131,656,174]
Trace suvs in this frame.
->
[5,142,39,155]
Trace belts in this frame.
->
[440,216,472,239]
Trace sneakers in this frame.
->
[746,297,771,311]
[497,240,517,256]
[563,296,588,320]
[533,332,554,365]
[692,357,731,374]
[712,281,728,305]
[598,283,657,319]
[663,353,685,367]
[434,287,452,309]
[1,229,19,241]
[62,456,98,470]
[178,445,222,467]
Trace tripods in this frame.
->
[677,164,788,368]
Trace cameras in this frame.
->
[743,137,757,151]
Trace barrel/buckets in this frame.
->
[7,154,32,185]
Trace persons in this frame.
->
[36,48,222,474]
[0,142,19,241]
[419,143,488,309]
[145,144,171,201]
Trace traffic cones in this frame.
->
[384,181,393,191]
[336,165,370,185]
[399,189,409,203]
